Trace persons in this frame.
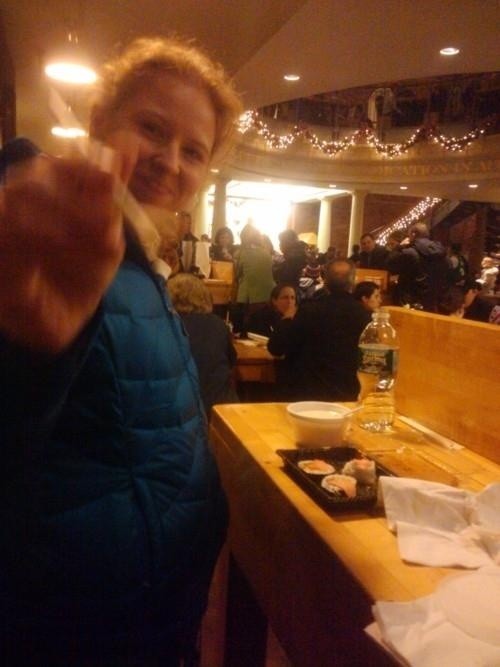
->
[157,209,500,402]
[2,34,241,661]
[1,137,42,178]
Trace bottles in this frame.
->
[355,305,399,431]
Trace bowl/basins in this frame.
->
[285,401,352,450]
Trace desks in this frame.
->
[159,245,500,384]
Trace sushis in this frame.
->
[297,457,375,499]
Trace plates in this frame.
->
[276,444,398,508]
